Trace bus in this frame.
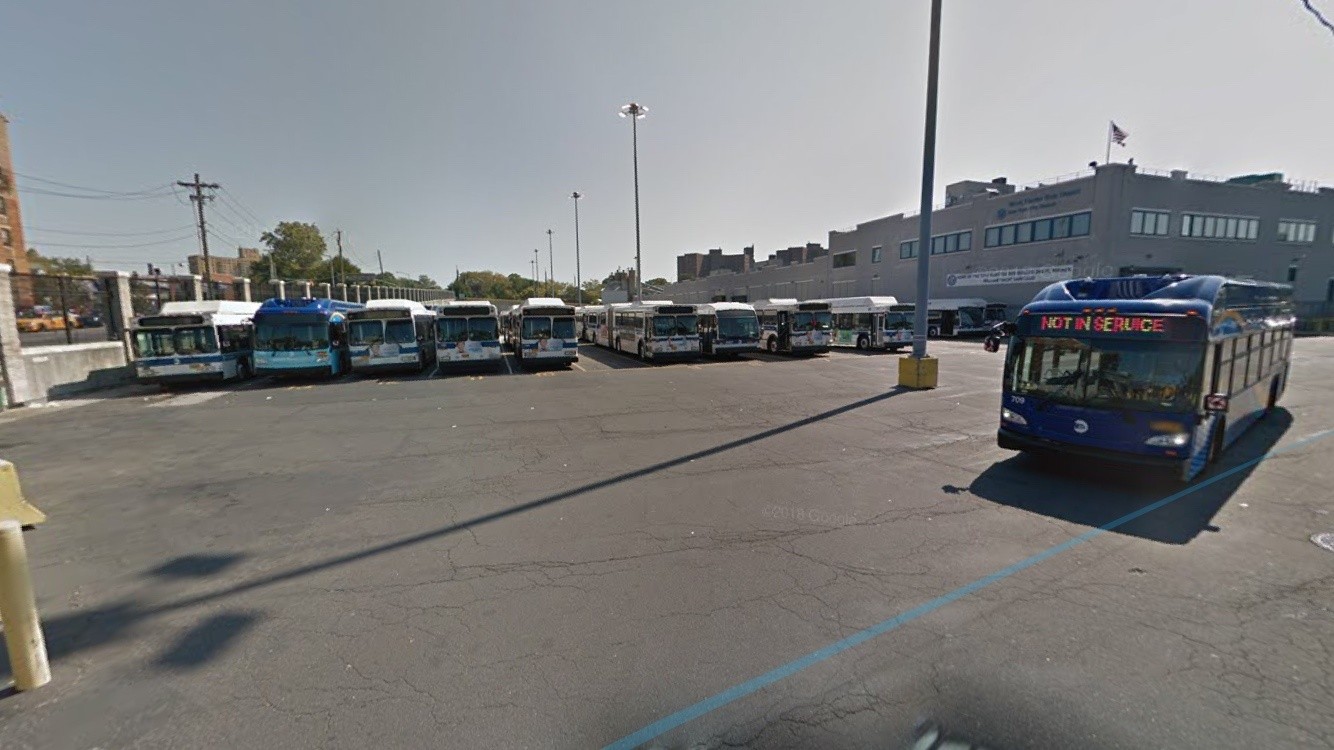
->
[344,298,438,374]
[433,301,504,372]
[582,300,704,362]
[574,306,584,340]
[984,275,1304,488]
[131,299,264,388]
[749,298,834,356]
[930,299,988,343]
[247,296,368,381]
[692,302,764,361]
[825,295,917,352]
[504,298,580,366]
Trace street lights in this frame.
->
[535,248,540,297]
[619,102,650,305]
[531,260,536,296]
[571,191,584,308]
[546,228,555,297]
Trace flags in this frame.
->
[1112,123,1128,146]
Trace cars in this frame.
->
[16,303,108,334]
